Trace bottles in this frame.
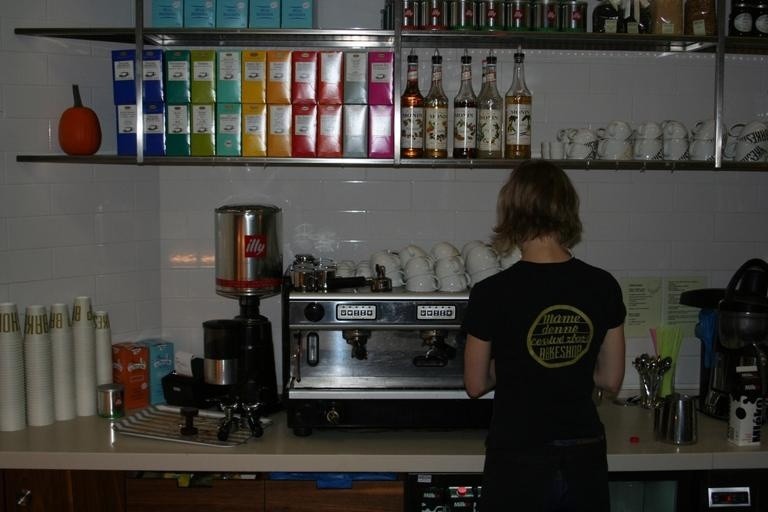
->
[290,253,335,292]
[401,48,533,160]
[389,1,588,33]
[591,0,767,41]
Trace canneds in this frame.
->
[96,384,124,421]
[728,1,768,38]
[401,0,587,34]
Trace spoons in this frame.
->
[633,354,674,411]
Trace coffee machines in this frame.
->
[157,199,284,445]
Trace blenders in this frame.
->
[676,255,768,427]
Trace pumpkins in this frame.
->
[60,84,101,155]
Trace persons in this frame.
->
[457,161,627,511]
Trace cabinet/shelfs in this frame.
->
[13,0,767,171]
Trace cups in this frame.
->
[337,240,522,294]
[0,295,127,433]
[540,119,768,161]
[652,393,699,447]
[637,369,665,410]
